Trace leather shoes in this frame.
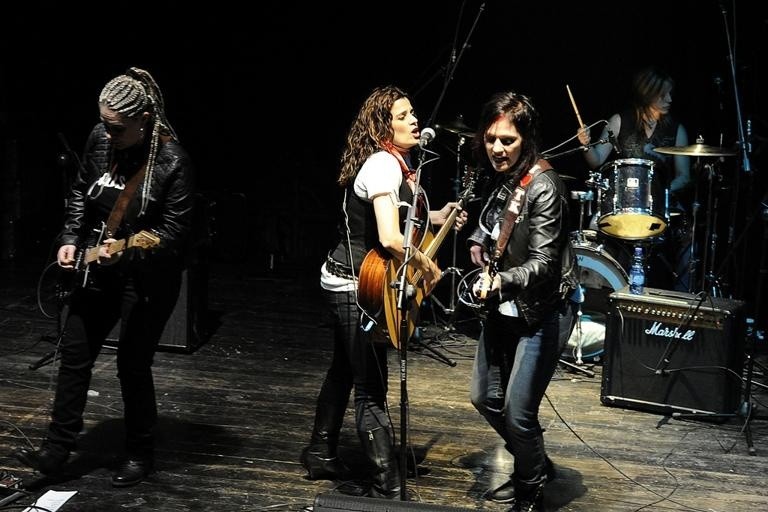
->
[14,446,66,483]
[113,457,157,488]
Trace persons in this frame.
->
[577,68,704,293]
[14,66,196,486]
[462,91,581,511]
[299,86,468,501]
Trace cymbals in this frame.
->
[653,144,737,156]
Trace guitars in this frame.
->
[470,250,493,301]
[46,221,163,277]
[359,164,485,351]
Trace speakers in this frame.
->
[312,491,488,512]
[102,265,215,353]
[602,284,744,423]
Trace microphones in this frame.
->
[603,122,622,154]
[419,127,437,150]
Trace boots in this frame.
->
[359,425,401,500]
[484,431,557,511]
[301,389,351,479]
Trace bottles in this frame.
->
[628,247,644,294]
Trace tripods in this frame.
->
[416,193,482,342]
[671,250,768,458]
[26,309,81,372]
[407,210,458,366]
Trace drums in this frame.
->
[594,158,666,240]
[559,230,632,361]
